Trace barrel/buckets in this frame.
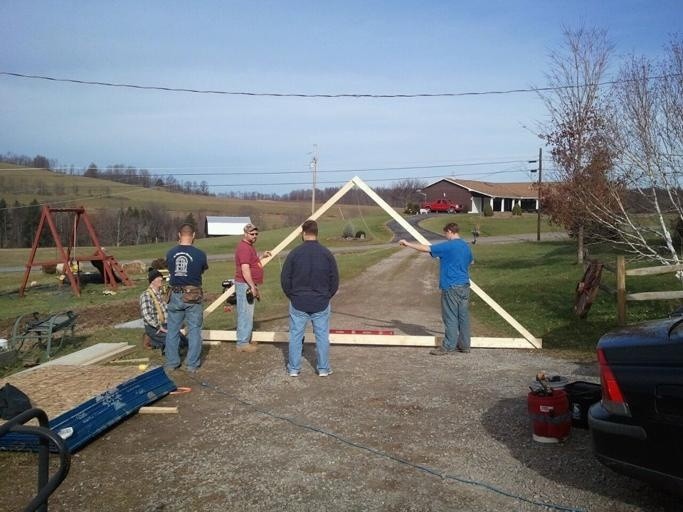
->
[527,390,572,444]
[530,375,569,391]
[565,381,602,428]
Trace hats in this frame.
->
[244,223,258,233]
[149,269,163,283]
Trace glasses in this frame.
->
[248,233,258,236]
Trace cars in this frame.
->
[587,315,682,489]
[423,199,465,213]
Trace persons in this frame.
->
[233,222,271,354]
[137,267,190,354]
[162,224,209,370]
[395,219,477,357]
[279,218,339,378]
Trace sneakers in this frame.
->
[318,368,334,377]
[144,335,153,350]
[456,345,470,354]
[429,347,455,355]
[235,343,257,353]
[290,371,300,377]
